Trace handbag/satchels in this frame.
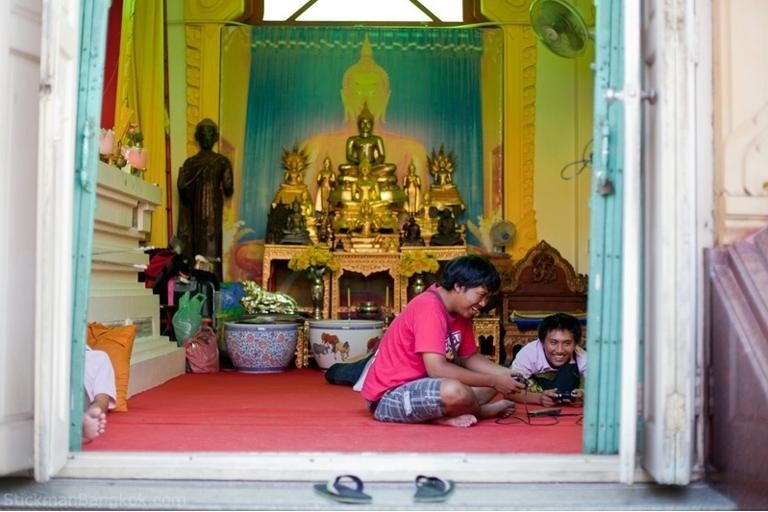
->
[183,326,220,374]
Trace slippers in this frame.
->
[313,476,372,504]
[413,476,455,503]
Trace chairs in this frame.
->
[502,240,588,366]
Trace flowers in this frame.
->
[287,243,344,279]
[397,250,440,278]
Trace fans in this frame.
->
[529,0,595,59]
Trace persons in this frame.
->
[177,118,233,290]
[361,256,526,429]
[272,45,466,246]
[503,313,586,407]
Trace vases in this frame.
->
[413,273,427,295]
[308,267,326,321]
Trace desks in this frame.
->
[400,246,488,312]
[261,244,329,319]
[330,252,401,319]
